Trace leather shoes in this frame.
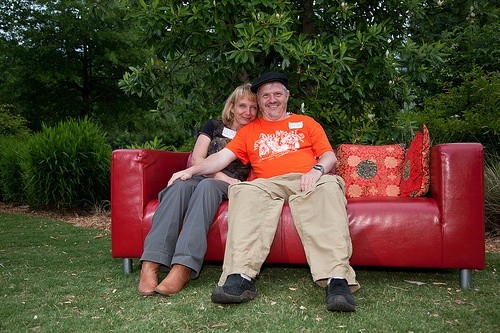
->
[138,260,159,296]
[154,263,192,296]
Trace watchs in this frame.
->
[313,165,325,174]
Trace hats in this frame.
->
[250,73,290,93]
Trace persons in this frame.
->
[167,71,357,312]
[137,82,259,298]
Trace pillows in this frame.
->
[335,144,404,197]
[398,125,431,198]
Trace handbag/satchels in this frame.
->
[208,137,250,180]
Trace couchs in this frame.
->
[111,124,486,289]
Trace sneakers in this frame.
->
[211,273,256,304]
[326,277,355,312]
